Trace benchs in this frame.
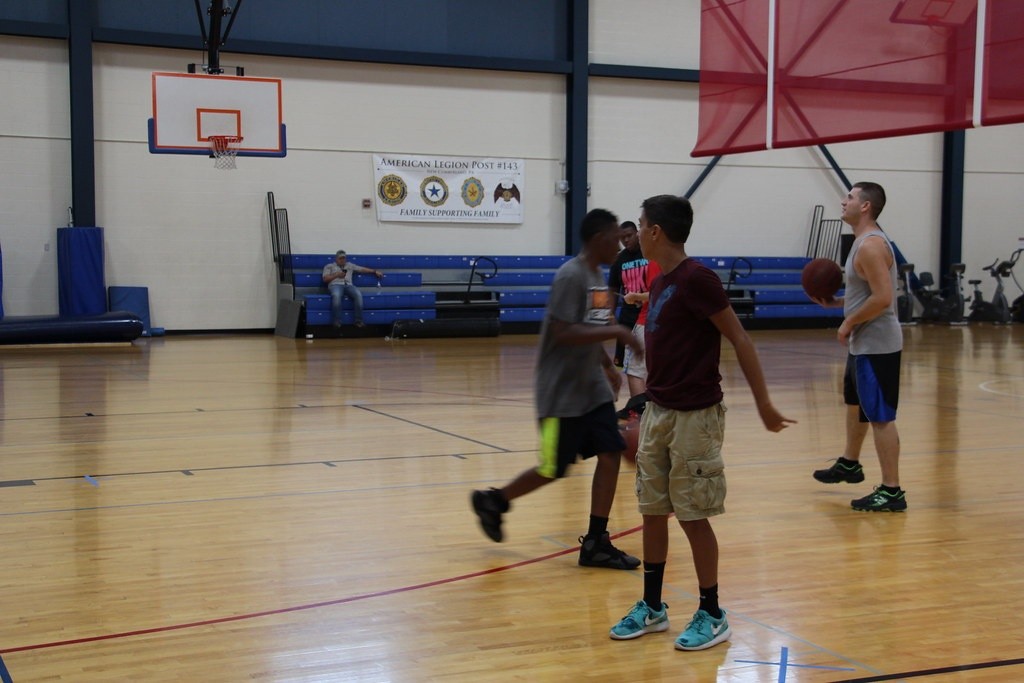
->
[290,255,849,325]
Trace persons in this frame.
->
[804,181,907,512]
[471,208,641,569]
[322,250,382,328]
[609,194,797,651]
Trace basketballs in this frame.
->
[800,256,845,299]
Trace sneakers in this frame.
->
[577,531,642,569]
[472,488,510,542]
[813,458,865,483]
[610,600,670,639]
[674,607,732,650]
[851,484,908,512]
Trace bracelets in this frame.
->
[374,270,376,273]
